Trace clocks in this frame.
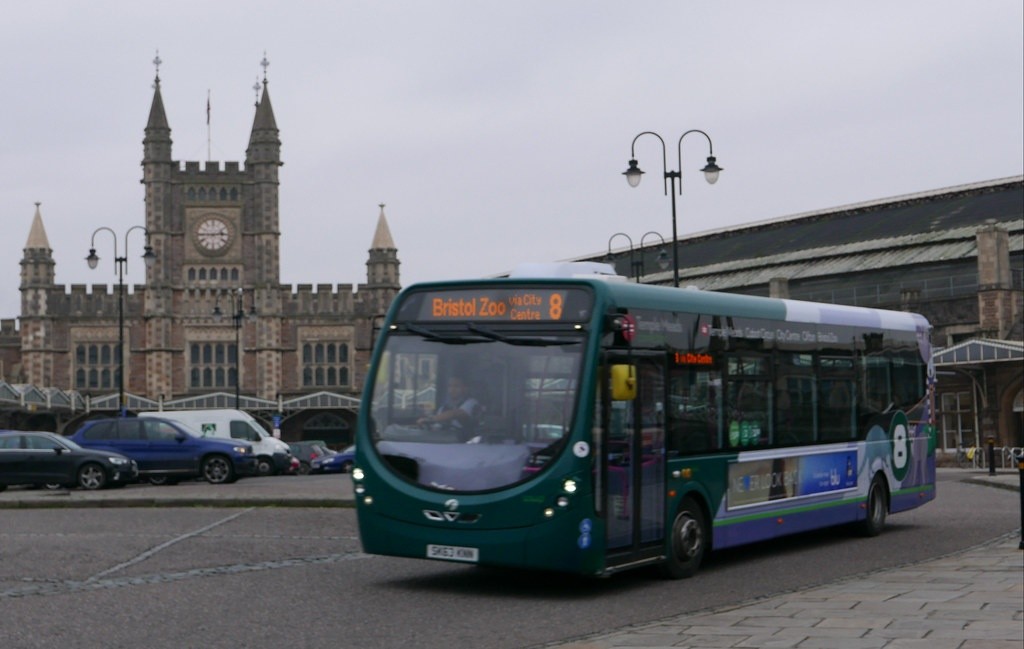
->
[188,214,237,258]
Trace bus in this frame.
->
[351,264,940,585]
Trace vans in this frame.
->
[134,407,291,475]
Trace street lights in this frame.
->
[211,287,259,408]
[621,128,723,286]
[88,225,155,416]
[605,230,674,287]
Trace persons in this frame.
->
[417,372,479,429]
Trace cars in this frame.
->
[320,445,360,475]
[0,427,138,491]
[282,437,325,475]
[71,416,253,485]
